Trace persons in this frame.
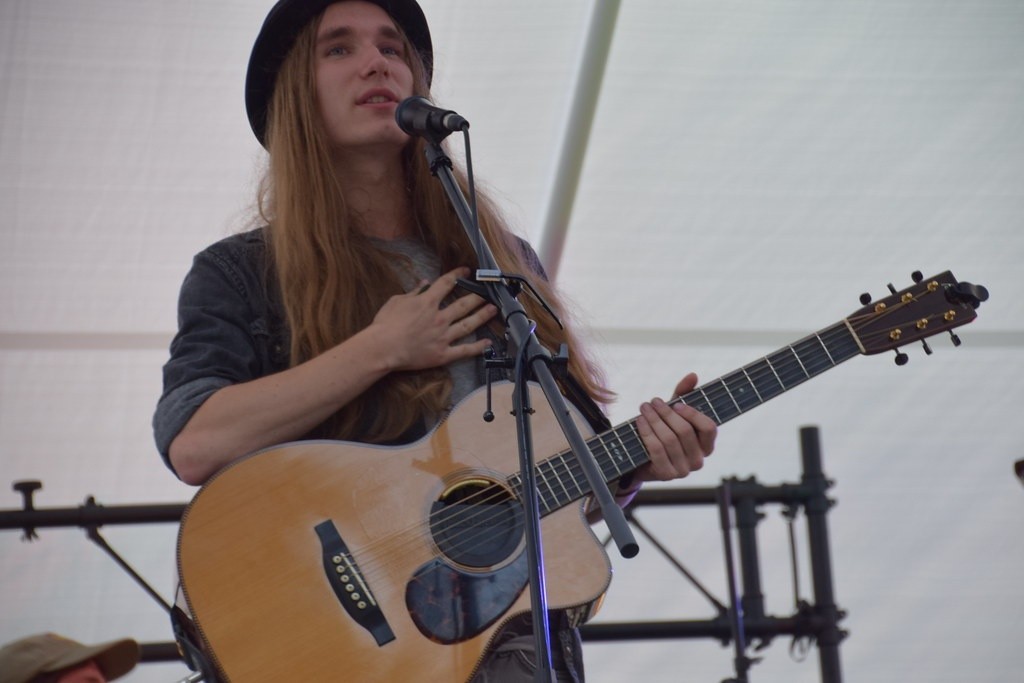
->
[153,0,718,683]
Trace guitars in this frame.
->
[174,265,993,683]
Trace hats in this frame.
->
[0,633,139,683]
[245,0,433,151]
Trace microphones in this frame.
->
[394,96,470,137]
